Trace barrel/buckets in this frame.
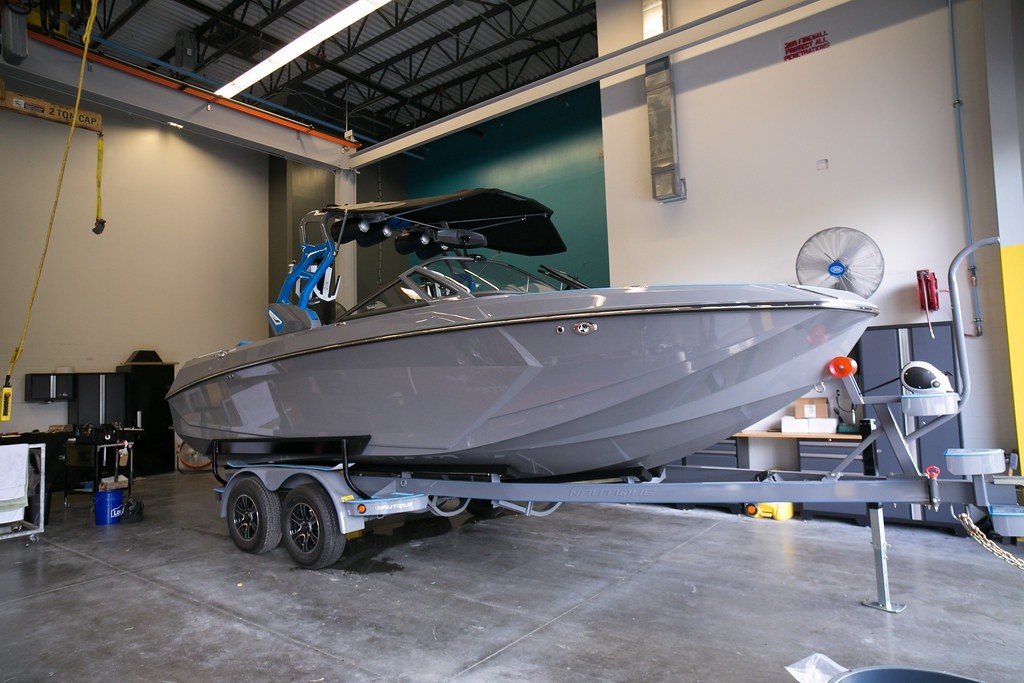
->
[91,489,123,525]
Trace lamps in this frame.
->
[212,0,392,100]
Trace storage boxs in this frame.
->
[794,397,831,418]
[781,414,839,435]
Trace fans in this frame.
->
[795,225,885,300]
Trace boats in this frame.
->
[169,187,883,481]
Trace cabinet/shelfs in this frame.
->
[115,350,176,478]
[24,373,75,404]
[681,430,864,471]
[63,442,135,507]
[68,371,126,426]
